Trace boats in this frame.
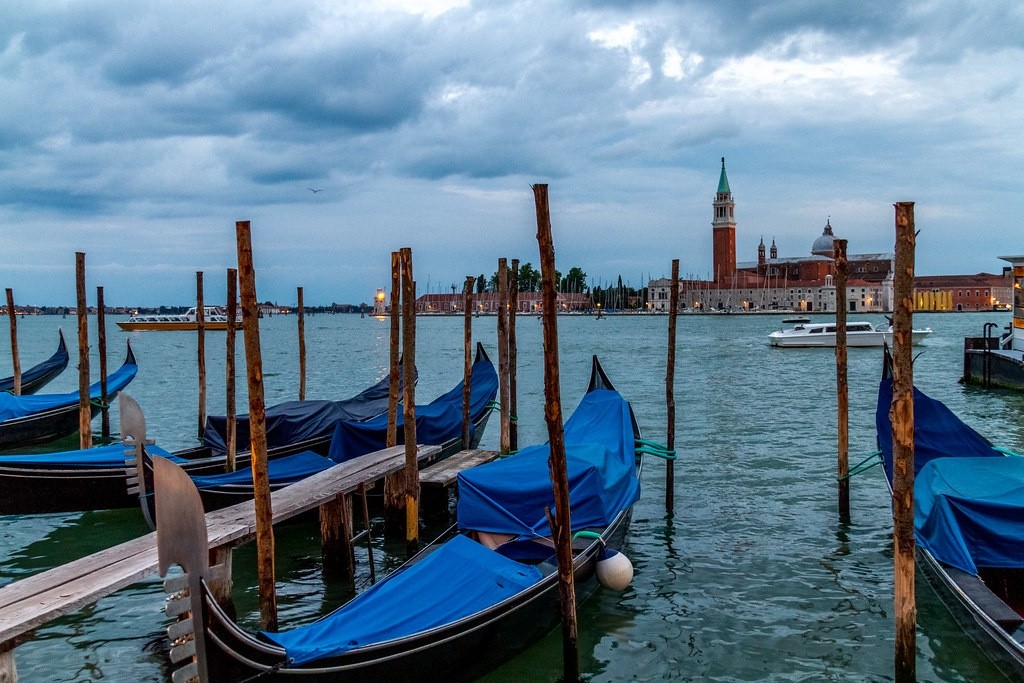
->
[0,338,138,446]
[198,355,644,683]
[0,327,69,395]
[782,316,811,323]
[115,306,244,330]
[766,322,932,348]
[140,341,499,508]
[875,341,1024,683]
[0,353,418,501]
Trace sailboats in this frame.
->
[379,263,798,313]
